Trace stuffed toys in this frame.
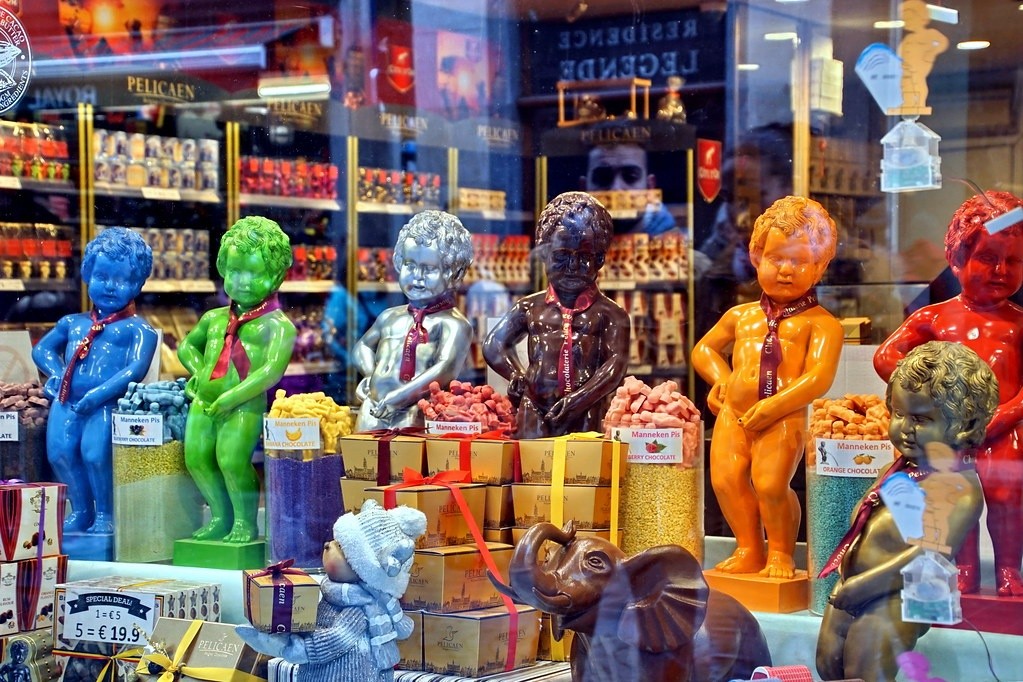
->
[234,499,428,682]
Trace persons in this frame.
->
[815,340,999,682]
[176,216,297,542]
[691,196,845,577]
[483,191,631,439]
[352,210,473,433]
[31,227,158,534]
[0,640,32,682]
[874,191,1023,596]
[703,122,860,283]
[579,124,675,235]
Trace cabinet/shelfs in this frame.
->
[542,148,694,410]
[353,135,455,404]
[453,145,543,296]
[88,103,234,382]
[0,104,87,335]
[232,120,357,405]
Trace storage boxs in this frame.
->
[340,432,628,679]
[0,121,689,398]
[0,480,319,682]
